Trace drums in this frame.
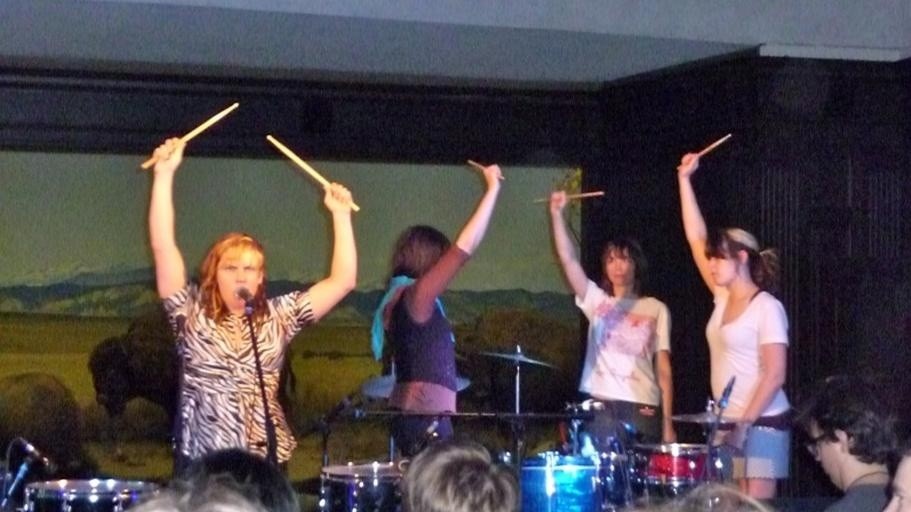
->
[625,441,714,486]
[319,464,406,512]
[516,446,606,512]
[25,479,158,512]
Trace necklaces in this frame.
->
[846,472,890,491]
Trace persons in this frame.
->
[882,431,911,512]
[548,191,676,445]
[784,375,902,512]
[398,439,522,512]
[182,449,301,512]
[627,480,781,512]
[147,137,357,482]
[371,157,502,456]
[677,152,794,501]
[123,462,269,512]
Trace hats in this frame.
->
[728,227,760,252]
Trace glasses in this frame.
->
[806,434,828,456]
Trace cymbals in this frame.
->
[672,410,737,426]
[480,350,558,372]
[362,371,470,400]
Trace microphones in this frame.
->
[17,436,58,475]
[717,376,736,409]
[563,399,605,414]
[237,284,256,311]
